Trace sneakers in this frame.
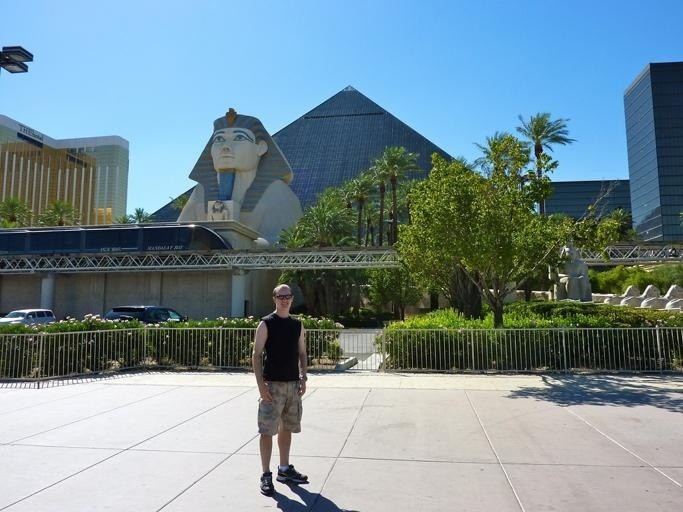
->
[276,465,308,483]
[260,472,275,496]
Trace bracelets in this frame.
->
[299,375,307,381]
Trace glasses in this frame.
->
[274,294,293,299]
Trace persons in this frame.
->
[177,108,303,249]
[210,201,228,220]
[252,284,308,495]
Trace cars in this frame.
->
[103,305,189,325]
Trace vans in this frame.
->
[1,308,57,329]
